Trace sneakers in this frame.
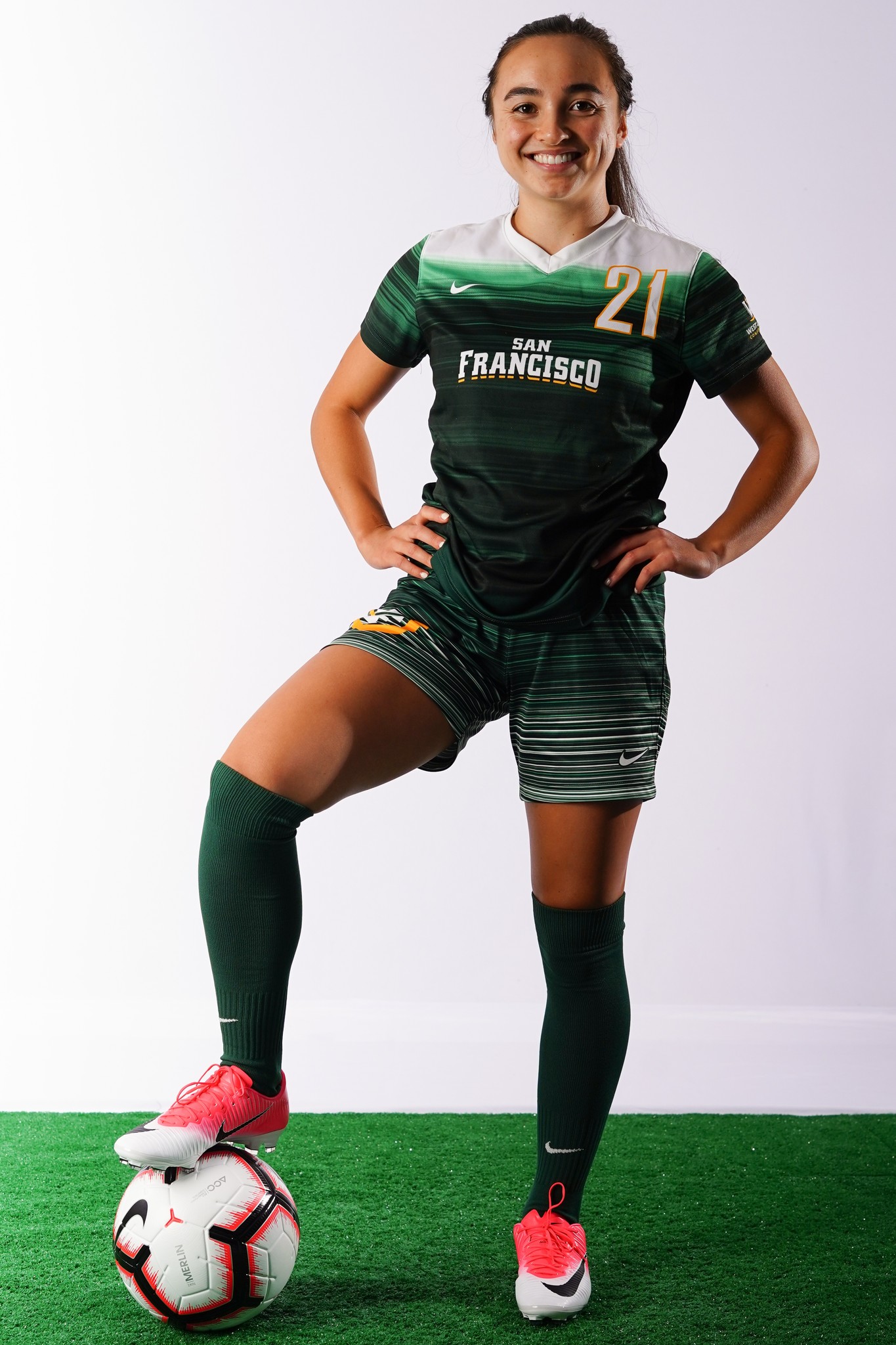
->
[512,1182,591,1324]
[115,1064,290,1170]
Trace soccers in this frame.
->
[112,1141,301,1334]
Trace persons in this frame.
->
[114,14,820,1321]
